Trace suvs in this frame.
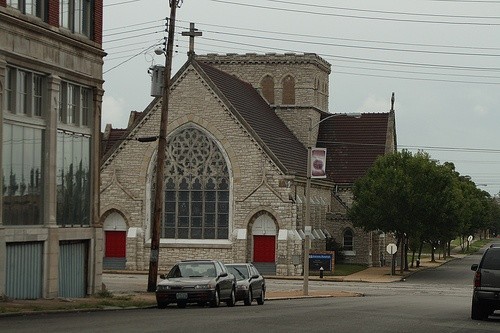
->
[472,241,500,323]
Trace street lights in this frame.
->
[302,111,364,296]
[147,47,175,295]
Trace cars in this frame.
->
[222,263,266,306]
[155,259,236,307]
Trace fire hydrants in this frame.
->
[320,266,324,278]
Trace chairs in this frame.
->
[183,267,215,277]
